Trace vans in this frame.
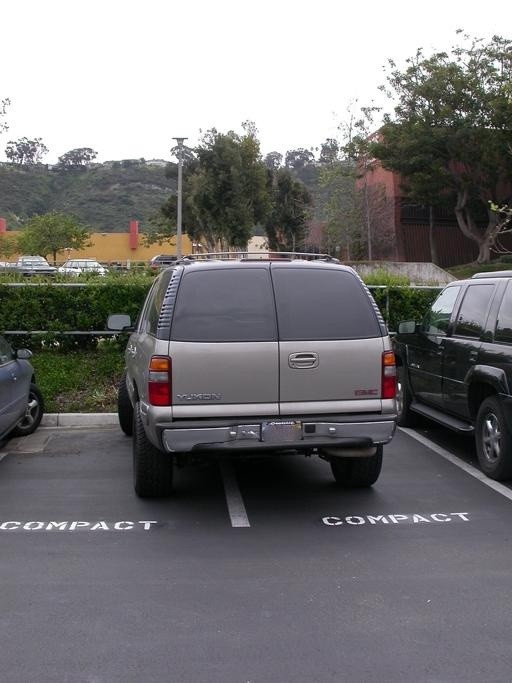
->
[118,252,404,498]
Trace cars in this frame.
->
[0,335,43,448]
[0,255,121,278]
[133,254,196,274]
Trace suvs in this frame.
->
[392,269,512,480]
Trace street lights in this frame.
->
[172,138,188,260]
